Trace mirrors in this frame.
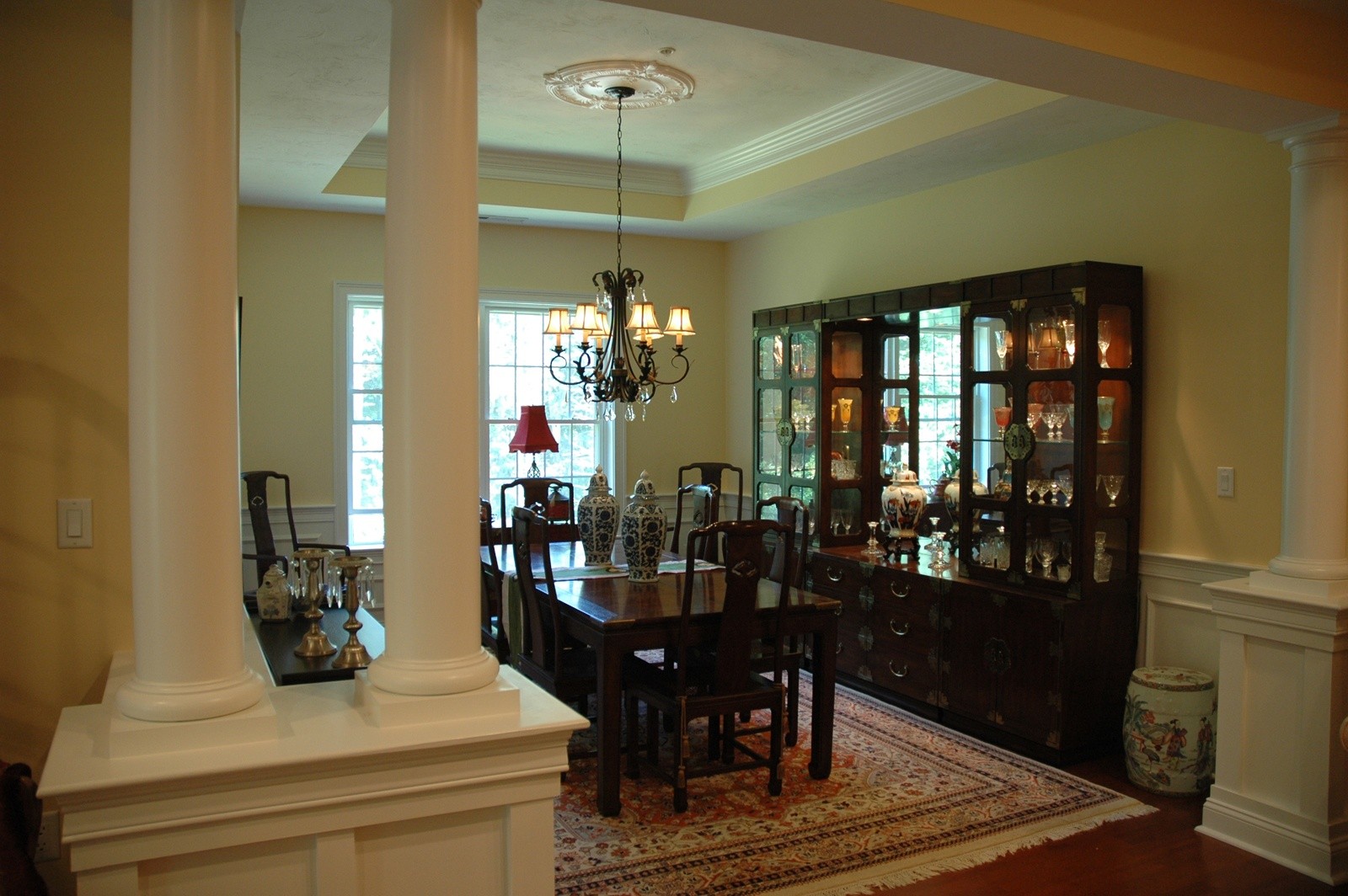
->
[871,323,1007,544]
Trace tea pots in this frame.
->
[257,565,292,620]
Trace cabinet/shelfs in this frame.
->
[751,261,1144,768]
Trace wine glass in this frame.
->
[832,508,853,536]
[1026,476,1123,508]
[991,397,1115,443]
[994,317,1113,370]
[792,411,816,431]
[838,399,854,433]
[1025,533,1073,582]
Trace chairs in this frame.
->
[241,471,350,612]
[987,461,1005,494]
[480,461,810,811]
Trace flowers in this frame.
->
[940,421,963,480]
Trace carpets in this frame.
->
[553,649,1160,896]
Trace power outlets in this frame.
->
[35,811,62,864]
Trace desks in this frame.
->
[923,497,953,540]
[492,517,580,543]
[481,540,843,815]
[247,604,386,687]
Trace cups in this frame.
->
[831,459,857,481]
[980,536,1010,571]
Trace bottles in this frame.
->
[1093,530,1112,583]
[578,466,620,566]
[622,471,665,583]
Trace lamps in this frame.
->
[542,87,697,421]
[508,405,559,478]
